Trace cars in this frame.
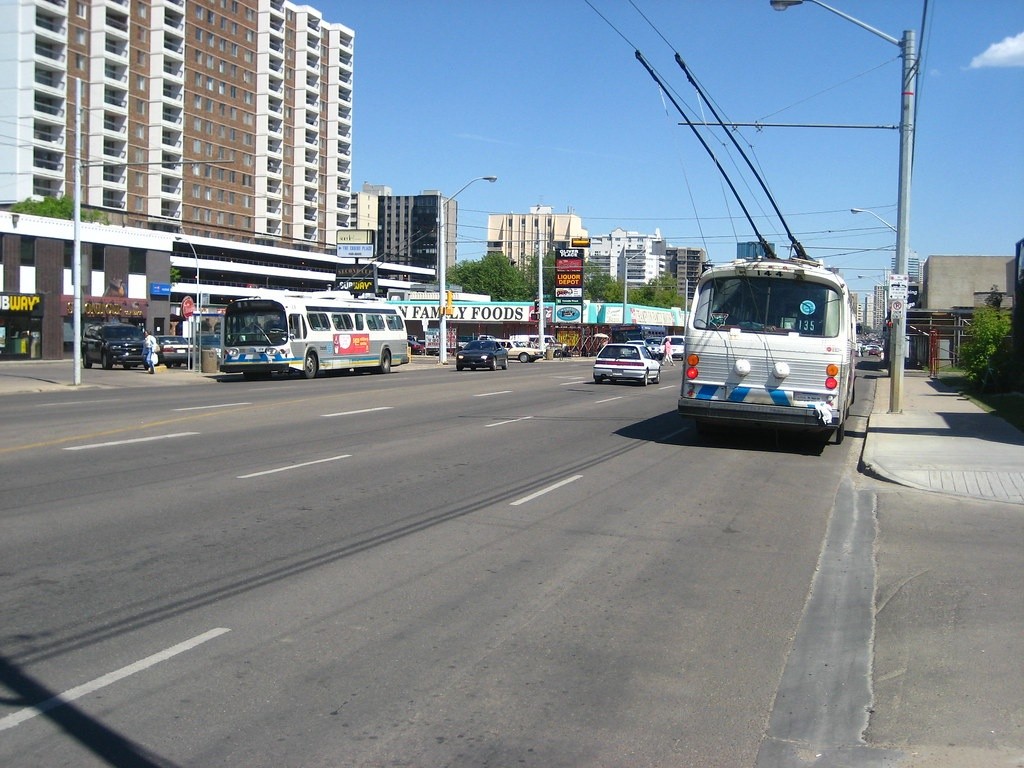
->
[593,343,661,385]
[153,336,198,369]
[857,337,884,353]
[494,339,543,363]
[455,340,508,371]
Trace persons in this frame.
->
[0,322,41,354]
[534,334,570,358]
[597,339,606,353]
[142,330,156,374]
[660,337,676,367]
[270,319,396,334]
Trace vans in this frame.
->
[409,337,423,354]
[82,322,147,370]
[629,335,685,360]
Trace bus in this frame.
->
[677,254,856,443]
[219,291,410,379]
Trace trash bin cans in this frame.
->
[203,350,217,372]
[546,349,553,360]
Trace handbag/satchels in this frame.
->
[154,344,161,353]
[151,352,158,365]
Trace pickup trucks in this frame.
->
[457,335,495,349]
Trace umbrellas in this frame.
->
[594,333,608,338]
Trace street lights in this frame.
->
[439,176,497,364]
[624,240,663,325]
[771,0,916,415]
[175,236,202,372]
[858,275,888,324]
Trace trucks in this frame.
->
[511,335,568,359]
